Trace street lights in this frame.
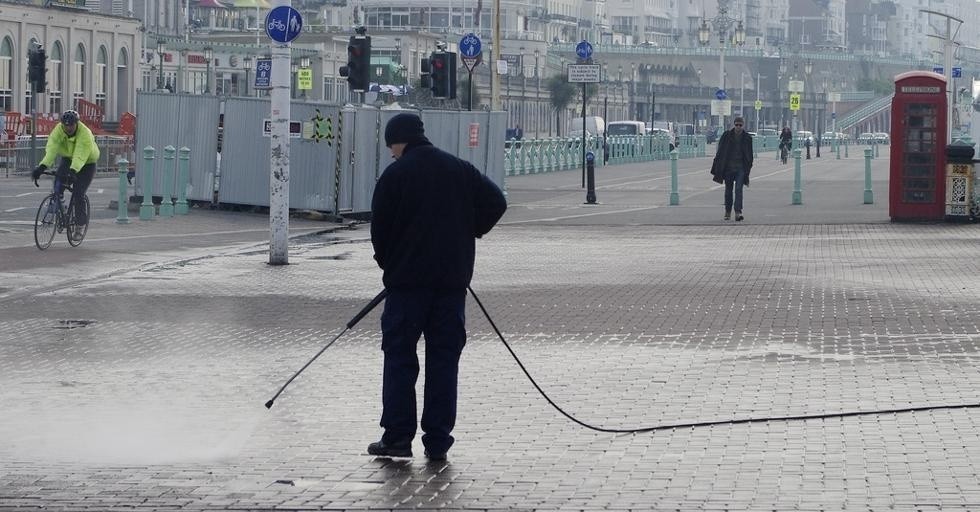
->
[697,0,746,140]
[243,54,253,96]
[400,66,408,101]
[156,39,167,89]
[299,56,310,98]
[202,46,214,94]
[697,57,851,134]
[375,65,383,99]
[291,62,300,99]
[394,36,402,69]
[602,60,652,123]
[488,42,569,139]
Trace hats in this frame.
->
[386,113,424,146]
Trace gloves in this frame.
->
[66,169,79,184]
[33,164,45,178]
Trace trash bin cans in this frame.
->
[944,140,975,222]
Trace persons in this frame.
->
[366,113,508,462]
[780,126,792,159]
[513,124,522,148]
[31,109,100,240]
[711,116,753,221]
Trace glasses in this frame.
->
[735,124,742,126]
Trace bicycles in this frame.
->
[32,168,90,250]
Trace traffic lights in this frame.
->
[431,51,451,101]
[37,49,49,93]
[347,36,371,93]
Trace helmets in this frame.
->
[61,111,79,125]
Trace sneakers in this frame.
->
[735,211,743,221]
[368,440,414,457]
[72,224,86,241]
[724,209,731,220]
[424,447,447,460]
